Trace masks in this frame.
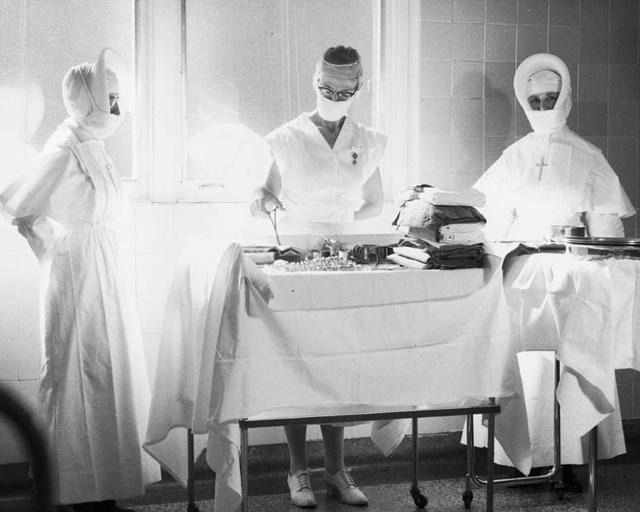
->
[80,111,122,139]
[523,110,566,132]
[316,93,353,121]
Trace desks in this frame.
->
[162,225,518,510]
[460,238,640,512]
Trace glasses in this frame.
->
[316,77,359,101]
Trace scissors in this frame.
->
[264,201,283,246]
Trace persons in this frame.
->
[5,62,162,511]
[470,52,636,494]
[249,47,383,507]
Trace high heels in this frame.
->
[322,468,369,506]
[287,466,318,507]
